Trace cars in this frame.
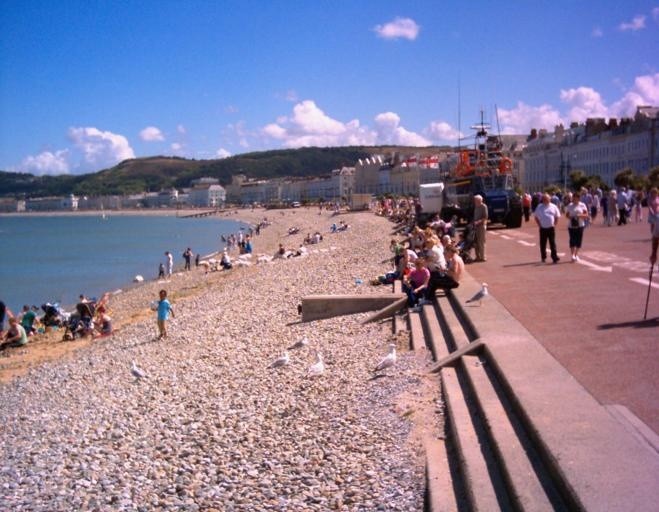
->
[291,201,301,208]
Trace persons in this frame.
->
[184,222,260,271]
[647,187,659,266]
[259,203,351,257]
[1,293,112,349]
[474,194,488,262]
[522,185,648,229]
[150,289,176,340]
[534,192,560,265]
[565,192,589,263]
[159,251,173,281]
[368,194,465,316]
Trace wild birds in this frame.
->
[128,360,147,381]
[465,282,489,307]
[266,350,290,369]
[308,352,324,378]
[373,343,397,375]
[298,303,302,315]
[287,334,307,350]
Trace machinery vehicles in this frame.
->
[440,109,524,230]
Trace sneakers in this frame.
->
[572,255,579,262]
[553,258,559,263]
